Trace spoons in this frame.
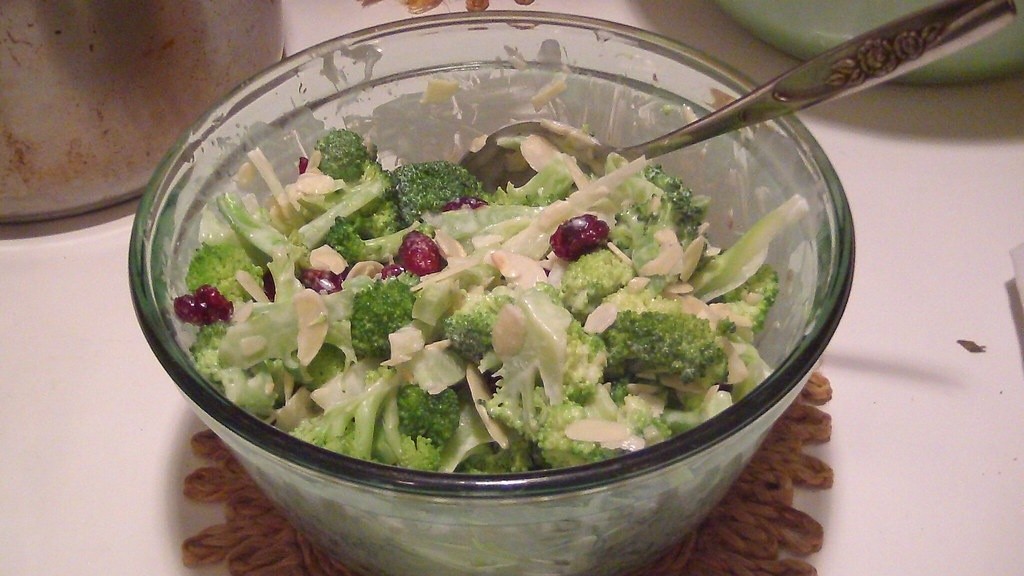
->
[454,0,1018,199]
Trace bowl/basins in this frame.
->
[710,0,1024,86]
[126,8,863,576]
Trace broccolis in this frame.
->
[189,129,814,477]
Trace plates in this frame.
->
[0,0,284,224]
